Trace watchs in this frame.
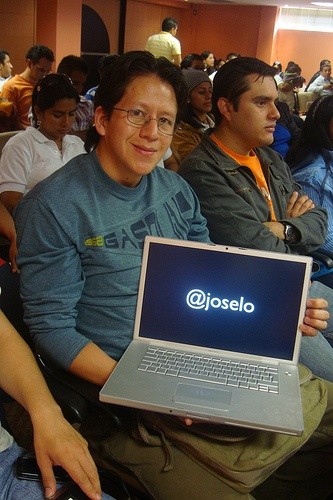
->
[279,221,295,241]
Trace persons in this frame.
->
[0,44,122,155]
[15,51,333,500]
[0,202,19,273]
[269,59,332,167]
[178,57,333,382]
[0,310,116,500]
[289,94,332,288]
[144,17,183,68]
[164,52,241,175]
[4,82,88,214]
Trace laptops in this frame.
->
[99,234,314,436]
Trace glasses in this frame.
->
[115,108,179,137]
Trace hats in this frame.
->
[183,70,212,96]
